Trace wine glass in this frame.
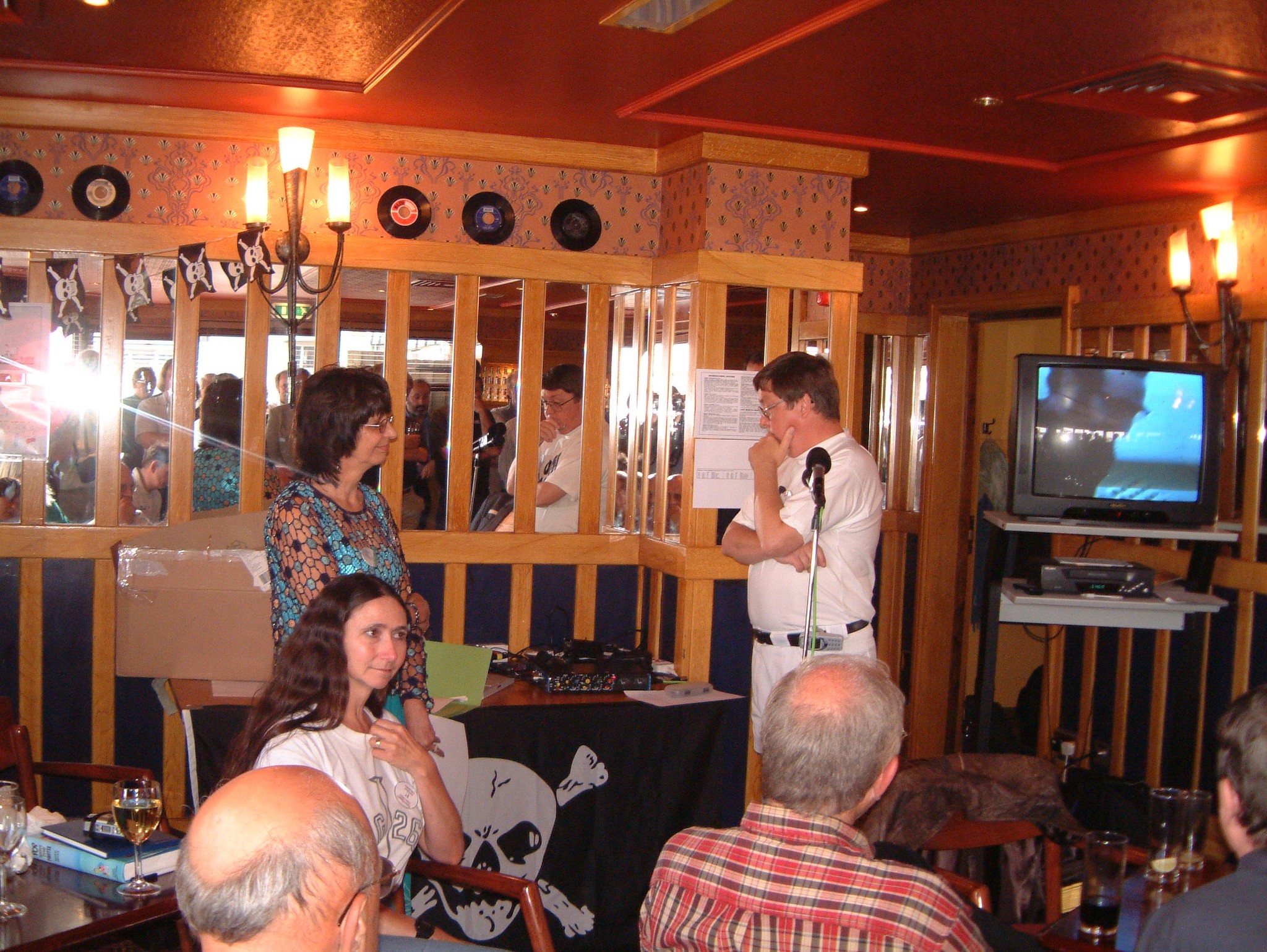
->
[0,780,29,920]
[111,779,162,896]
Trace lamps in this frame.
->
[247,126,352,410]
[1168,198,1251,453]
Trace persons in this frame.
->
[722,351,884,755]
[1035,428,1121,444]
[224,573,514,952]
[1035,367,1151,495]
[0,350,240,526]
[1134,683,1267,952]
[264,367,445,758]
[173,766,399,952]
[359,360,503,531]
[492,364,686,532]
[266,369,310,486]
[637,655,995,951]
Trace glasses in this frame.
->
[336,857,401,928]
[358,413,395,433]
[540,394,575,413]
[758,397,790,422]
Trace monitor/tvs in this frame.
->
[1006,351,1220,525]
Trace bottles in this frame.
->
[481,366,514,400]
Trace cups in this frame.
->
[1144,788,1189,885]
[1080,831,1129,936]
[407,422,421,436]
[1180,790,1213,872]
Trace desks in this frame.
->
[971,508,1239,912]
[0,813,203,952]
[412,644,746,952]
[853,750,1239,952]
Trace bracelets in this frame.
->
[406,602,420,625]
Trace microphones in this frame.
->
[779,486,787,495]
[801,447,833,506]
[473,422,507,451]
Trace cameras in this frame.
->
[82,813,127,840]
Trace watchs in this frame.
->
[415,915,434,939]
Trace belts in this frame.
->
[752,620,871,647]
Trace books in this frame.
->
[26,819,182,884]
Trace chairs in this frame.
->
[0,694,173,836]
[405,855,553,952]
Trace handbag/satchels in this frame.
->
[75,453,95,483]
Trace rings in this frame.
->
[433,745,438,753]
[376,740,380,749]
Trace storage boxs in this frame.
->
[113,508,275,683]
